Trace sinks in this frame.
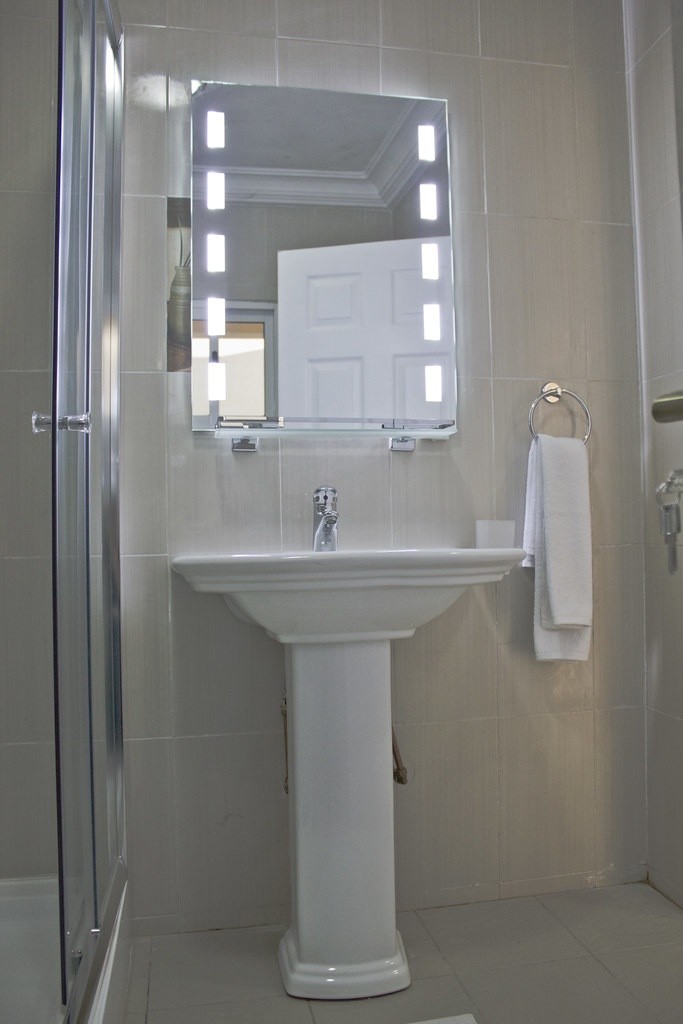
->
[172,550,526,645]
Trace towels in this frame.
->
[520,432,593,662]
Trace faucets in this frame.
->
[311,485,341,552]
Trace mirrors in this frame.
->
[188,80,459,435]
